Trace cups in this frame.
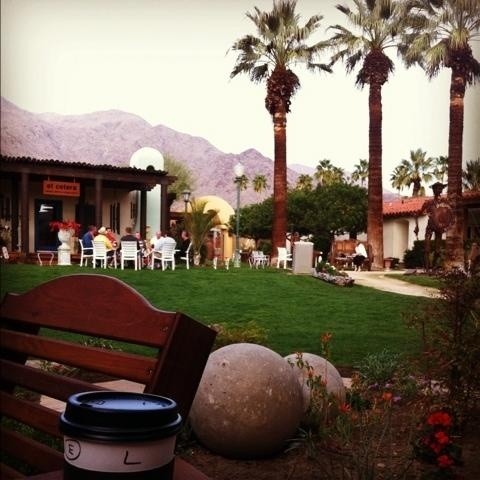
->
[57,389,185,480]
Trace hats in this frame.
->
[98,227,107,234]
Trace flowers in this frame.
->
[49,218,81,236]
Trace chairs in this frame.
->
[250,246,293,270]
[77,237,193,272]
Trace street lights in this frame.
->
[231,161,246,271]
[182,186,190,218]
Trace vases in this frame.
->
[57,228,71,249]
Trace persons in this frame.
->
[351,238,368,273]
[81,223,192,271]
